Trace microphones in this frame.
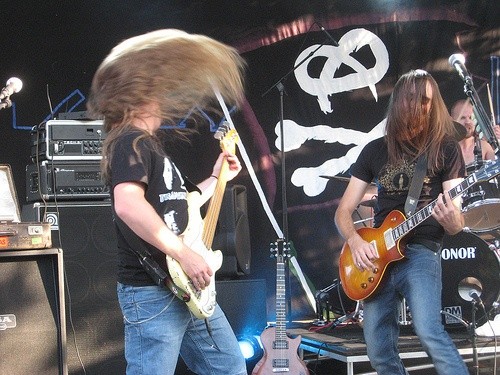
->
[449,53,472,83]
[317,22,339,47]
[469,289,484,308]
[0,76,23,100]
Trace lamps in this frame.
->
[235,334,263,375]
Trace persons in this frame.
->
[333,69,470,375]
[450,99,500,170]
[88,28,249,375]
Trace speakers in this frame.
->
[0,201,129,375]
[200,183,251,280]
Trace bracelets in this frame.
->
[211,175,217,180]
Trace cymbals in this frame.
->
[319,173,352,183]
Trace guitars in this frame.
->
[165,121,239,319]
[339,160,499,301]
[251,238,309,375]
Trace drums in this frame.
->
[440,235,500,332]
[452,159,500,233]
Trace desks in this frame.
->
[0,246,70,375]
